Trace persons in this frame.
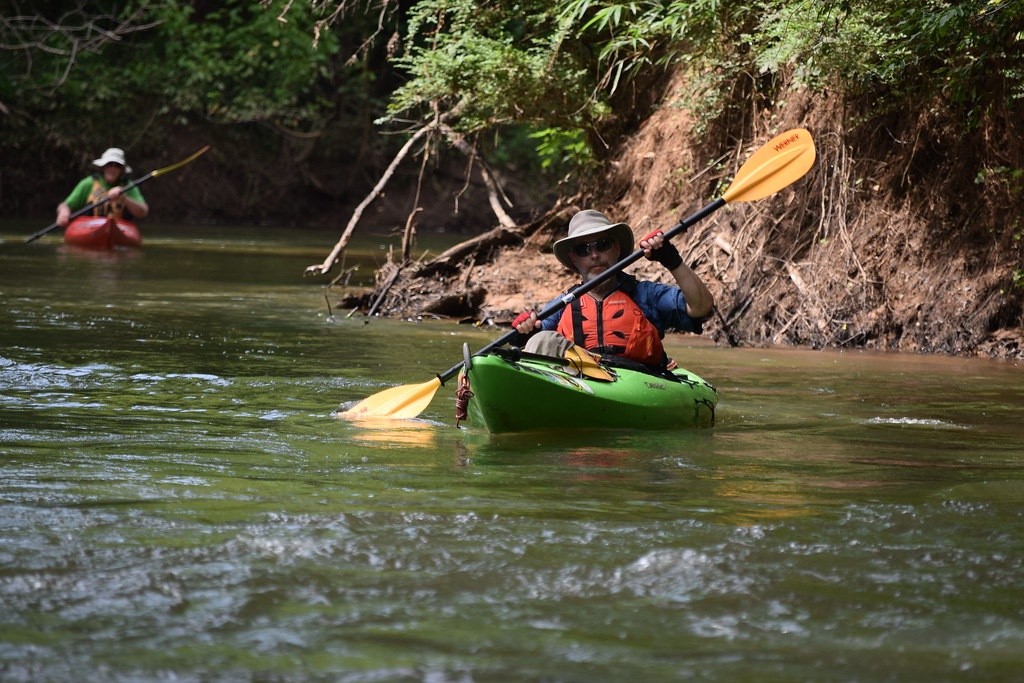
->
[510,209,715,373]
[56,148,149,227]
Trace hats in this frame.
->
[553,210,634,274]
[94,148,132,174]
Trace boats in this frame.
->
[457,342,718,434]
[64,213,141,250]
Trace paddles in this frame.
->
[23,145,213,244]
[342,129,816,422]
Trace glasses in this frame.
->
[573,237,613,257]
[108,163,123,168]
